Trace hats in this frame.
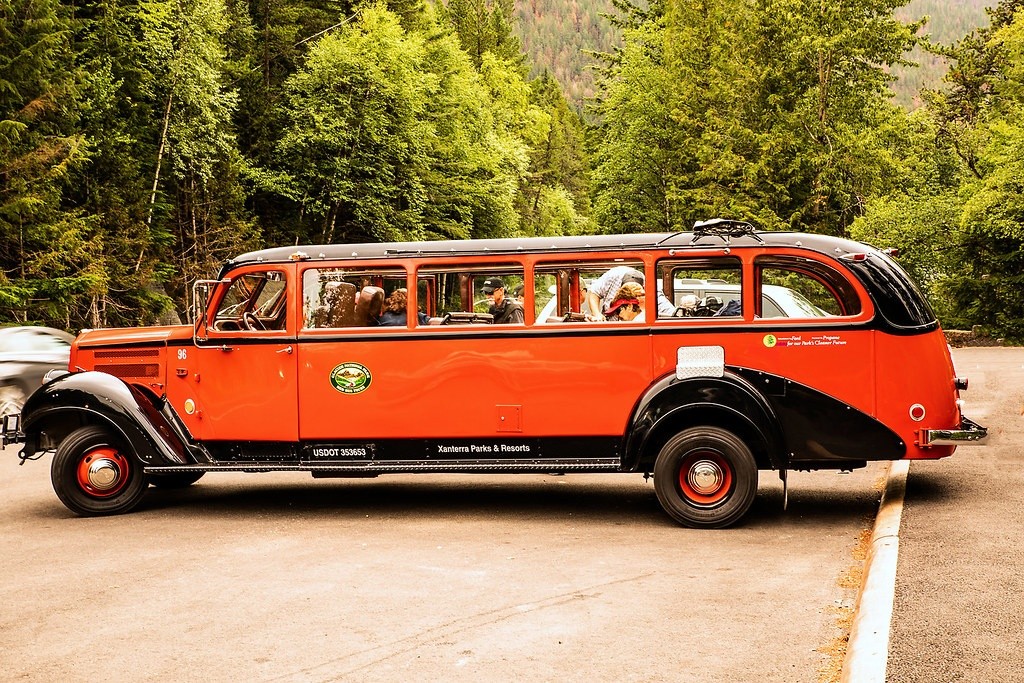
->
[605,299,639,314]
[480,277,505,293]
[580,277,588,291]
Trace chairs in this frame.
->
[314,283,385,328]
[441,307,687,325]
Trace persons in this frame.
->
[379,287,444,326]
[576,265,677,322]
[482,277,525,323]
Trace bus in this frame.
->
[16,219,988,529]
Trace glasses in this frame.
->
[484,287,500,295]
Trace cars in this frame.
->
[0,322,78,434]
[534,276,833,319]
